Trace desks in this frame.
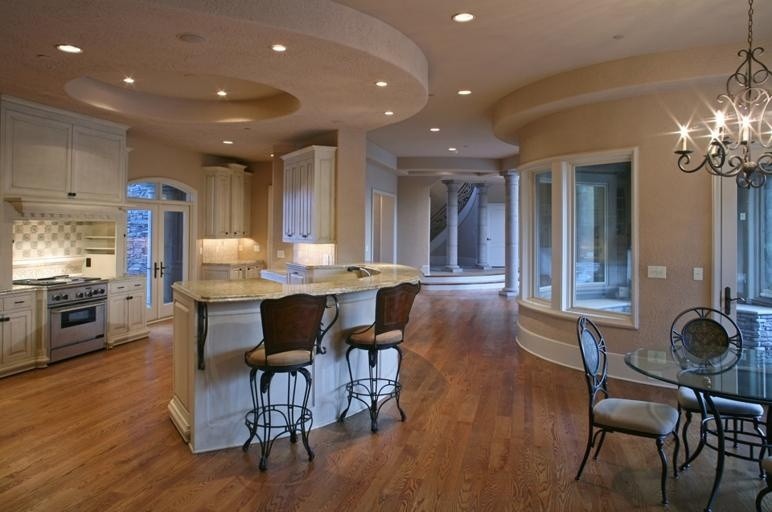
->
[624,346,772,511]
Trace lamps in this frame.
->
[674,0,772,188]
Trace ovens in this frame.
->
[46,301,106,350]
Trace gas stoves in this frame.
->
[11,273,109,290]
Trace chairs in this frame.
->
[756,456,771,512]
[670,307,766,479]
[243,293,340,471]
[576,316,680,504]
[338,280,420,434]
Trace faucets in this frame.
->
[347,266,370,276]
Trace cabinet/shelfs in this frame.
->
[82,220,117,276]
[203,260,266,281]
[245,172,254,237]
[280,144,336,244]
[287,263,348,286]
[0,284,50,380]
[225,163,247,237]
[199,167,229,238]
[105,276,150,350]
[0,93,131,202]
